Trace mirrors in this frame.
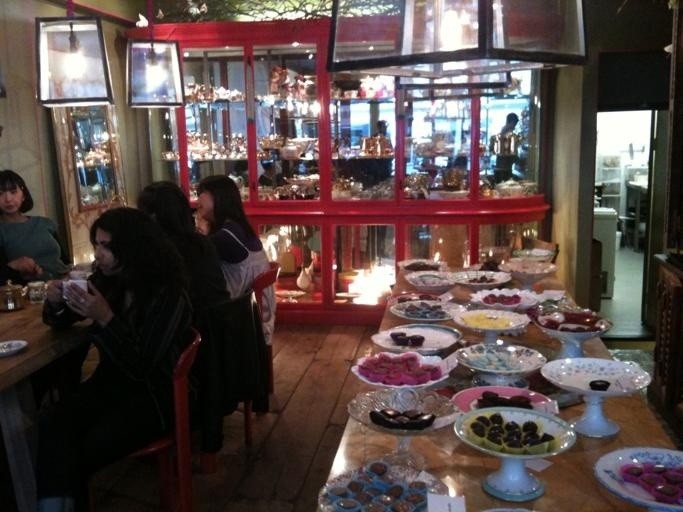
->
[66,103,125,212]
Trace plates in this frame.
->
[0,340,29,358]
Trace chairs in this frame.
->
[234,261,284,447]
[123,334,202,512]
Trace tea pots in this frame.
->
[274,139,302,161]
[0,279,27,312]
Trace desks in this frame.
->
[318,273,682,510]
[629,180,648,251]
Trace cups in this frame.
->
[63,280,89,300]
[28,281,45,304]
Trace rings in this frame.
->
[80,298,86,303]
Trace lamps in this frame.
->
[31,13,116,111]
[326,1,591,80]
[122,37,187,111]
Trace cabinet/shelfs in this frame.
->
[596,153,627,235]
[119,21,548,204]
[246,204,550,328]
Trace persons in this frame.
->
[259,155,281,185]
[447,130,467,168]
[192,174,276,416]
[488,113,522,184]
[228,160,249,181]
[136,180,245,452]
[35,206,194,512]
[374,120,391,140]
[1,169,73,284]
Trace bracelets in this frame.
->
[55,308,64,317]
